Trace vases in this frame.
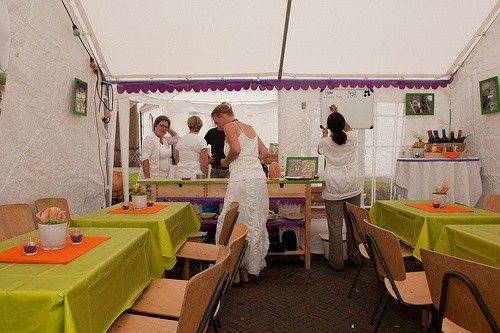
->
[432,192,447,208]
[132,194,148,210]
[413,147,425,157]
[39,223,68,250]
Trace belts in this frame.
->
[213,166,229,170]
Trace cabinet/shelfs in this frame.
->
[136,177,325,271]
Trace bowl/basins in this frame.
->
[443,151,462,158]
[425,142,466,153]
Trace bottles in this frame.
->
[440,129,449,143]
[457,129,463,143]
[433,130,440,143]
[448,130,457,142]
[428,130,435,143]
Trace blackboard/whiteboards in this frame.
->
[320,88,373,129]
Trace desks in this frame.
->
[70,203,201,270]
[393,158,482,207]
[0,228,167,333]
[435,225,500,268]
[367,200,500,262]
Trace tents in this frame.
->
[0,0,500,230]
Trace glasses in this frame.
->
[159,123,168,129]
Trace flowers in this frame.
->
[37,207,66,223]
[434,185,449,193]
[130,184,147,194]
[412,131,425,147]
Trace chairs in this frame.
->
[0,198,249,333]
[363,218,435,333]
[419,249,500,333]
[111,171,139,204]
[481,195,500,213]
[345,201,420,324]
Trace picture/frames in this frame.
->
[286,157,319,177]
[73,78,88,117]
[405,93,434,116]
[479,75,500,115]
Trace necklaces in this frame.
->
[232,119,238,122]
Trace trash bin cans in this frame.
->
[321,234,349,262]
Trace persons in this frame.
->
[175,116,209,180]
[77,86,85,113]
[137,115,180,191]
[204,126,230,178]
[210,102,270,285]
[317,104,362,271]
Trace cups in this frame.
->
[147,200,154,207]
[120,202,130,211]
[20,237,40,256]
[69,228,84,245]
[433,199,441,208]
